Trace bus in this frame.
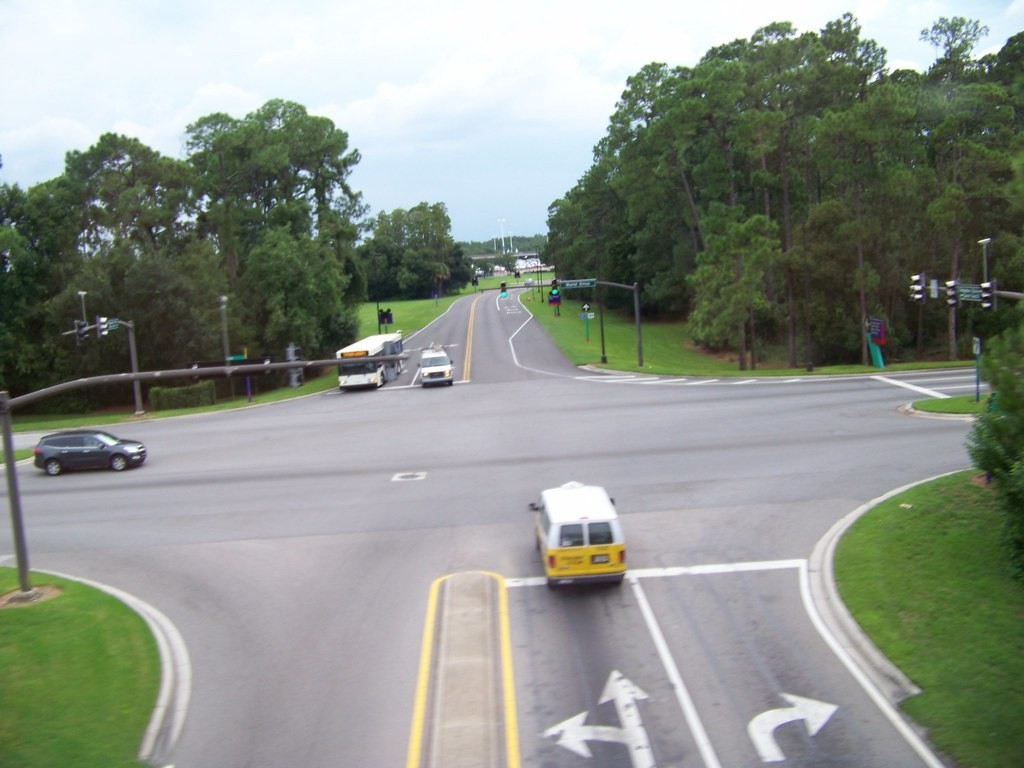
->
[335,330,404,390]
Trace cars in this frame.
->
[33,430,147,477]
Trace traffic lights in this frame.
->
[908,274,926,302]
[551,278,560,296]
[380,308,391,318]
[981,276,998,313]
[96,313,109,340]
[74,321,91,346]
[500,282,507,298]
[945,280,959,307]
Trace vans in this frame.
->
[527,482,627,591]
[417,348,454,388]
